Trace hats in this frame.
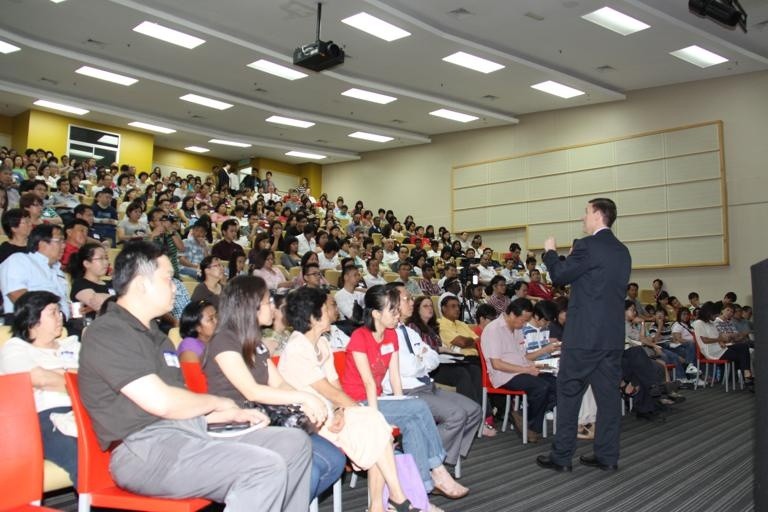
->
[355,226,367,233]
[350,242,361,247]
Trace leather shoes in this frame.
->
[580,454,619,471]
[536,454,573,471]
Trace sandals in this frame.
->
[387,497,422,512]
[431,472,470,500]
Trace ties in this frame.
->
[400,324,431,386]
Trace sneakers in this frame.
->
[677,378,709,386]
[744,376,754,384]
[509,410,538,442]
[480,425,498,437]
[649,380,686,404]
[686,365,703,374]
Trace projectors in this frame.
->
[292,40,344,72]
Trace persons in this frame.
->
[1,142,756,512]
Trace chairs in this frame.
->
[694,329,735,392]
[272,356,360,488]
[64,373,213,512]
[179,363,342,512]
[475,338,548,444]
[331,352,401,442]
[0,371,63,512]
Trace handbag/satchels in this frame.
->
[245,398,315,436]
[382,450,432,512]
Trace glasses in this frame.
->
[207,263,224,269]
[260,297,275,307]
[401,295,415,301]
[306,272,322,277]
